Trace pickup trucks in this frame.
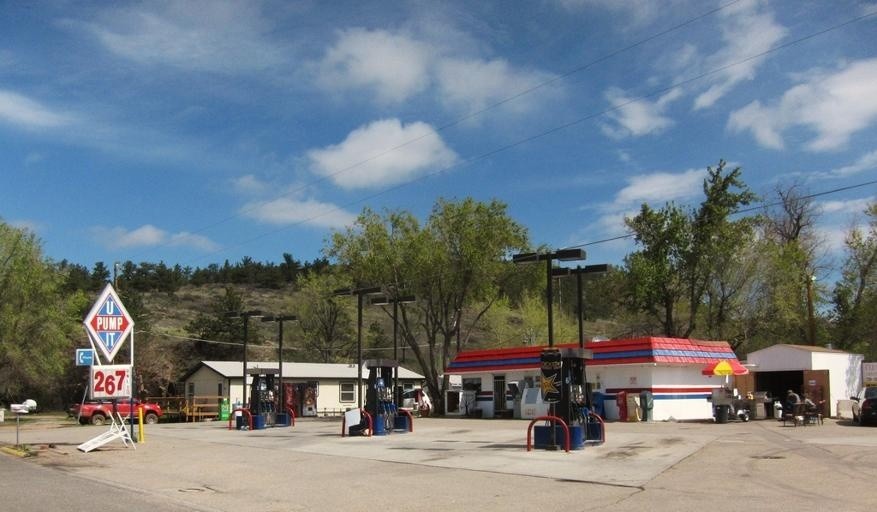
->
[68,394,163,426]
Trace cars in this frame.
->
[848,385,877,425]
[401,388,432,415]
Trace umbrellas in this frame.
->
[701,359,749,384]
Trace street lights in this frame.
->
[370,294,416,413]
[260,312,297,414]
[334,286,381,418]
[549,263,612,424]
[511,248,587,454]
[224,309,267,425]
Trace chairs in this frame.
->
[806,398,828,427]
[782,402,806,429]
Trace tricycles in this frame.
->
[706,387,753,424]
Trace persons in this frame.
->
[786,390,801,403]
[800,393,815,406]
